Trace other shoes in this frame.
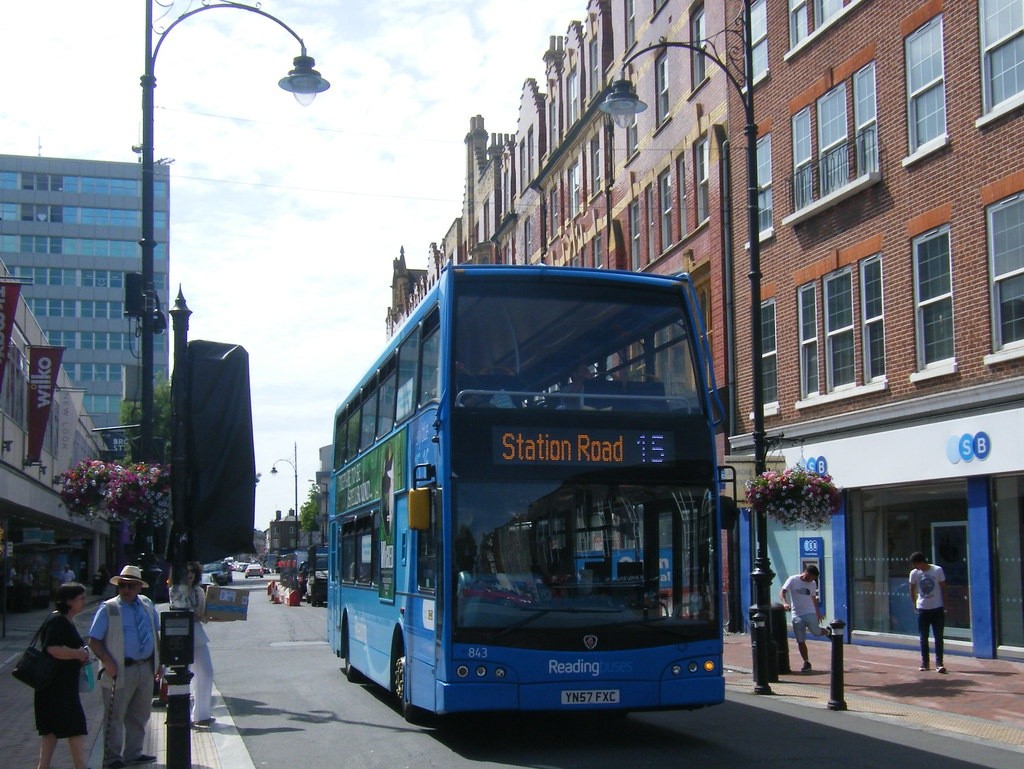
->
[826,626,833,642]
[800,662,812,671]
[124,754,157,764]
[919,663,929,671]
[193,718,215,725]
[107,760,128,769]
[935,666,947,673]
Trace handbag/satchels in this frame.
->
[11,612,71,690]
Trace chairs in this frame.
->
[470,367,670,414]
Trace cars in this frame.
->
[245,564,264,579]
[198,556,250,593]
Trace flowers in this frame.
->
[745,463,842,529]
[54,457,170,529]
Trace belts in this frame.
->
[133,656,152,665]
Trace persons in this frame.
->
[908,551,949,673]
[88,564,166,769]
[779,565,833,672]
[168,559,216,725]
[350,559,368,581]
[92,565,114,595]
[6,568,17,610]
[33,581,88,769]
[567,364,616,407]
[60,564,75,583]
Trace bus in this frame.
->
[326,260,741,723]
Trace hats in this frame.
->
[110,565,150,588]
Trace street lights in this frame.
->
[599,0,773,695]
[123,0,331,601]
[270,441,298,549]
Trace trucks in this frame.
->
[278,544,327,607]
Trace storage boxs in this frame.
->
[204,586,249,619]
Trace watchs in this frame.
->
[944,610,947,613]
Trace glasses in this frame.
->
[186,568,195,575]
[118,581,142,590]
